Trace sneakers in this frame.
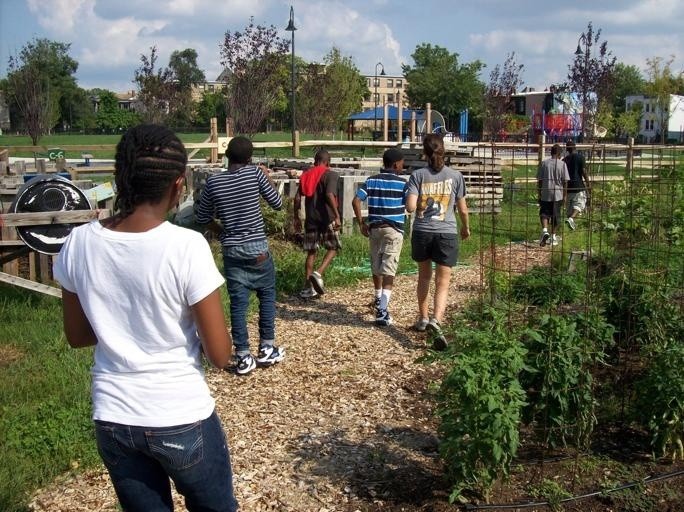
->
[256,345,285,366]
[416,319,429,331]
[551,241,558,247]
[237,354,256,374]
[540,232,550,247]
[301,289,318,299]
[425,319,448,350]
[565,218,575,230]
[374,297,381,308]
[376,309,393,325]
[310,273,325,294]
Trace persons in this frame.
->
[560,142,592,230]
[195,135,284,377]
[53,124,240,511]
[295,150,341,297]
[350,148,408,326]
[536,143,571,246]
[402,133,469,349]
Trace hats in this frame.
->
[383,149,404,161]
